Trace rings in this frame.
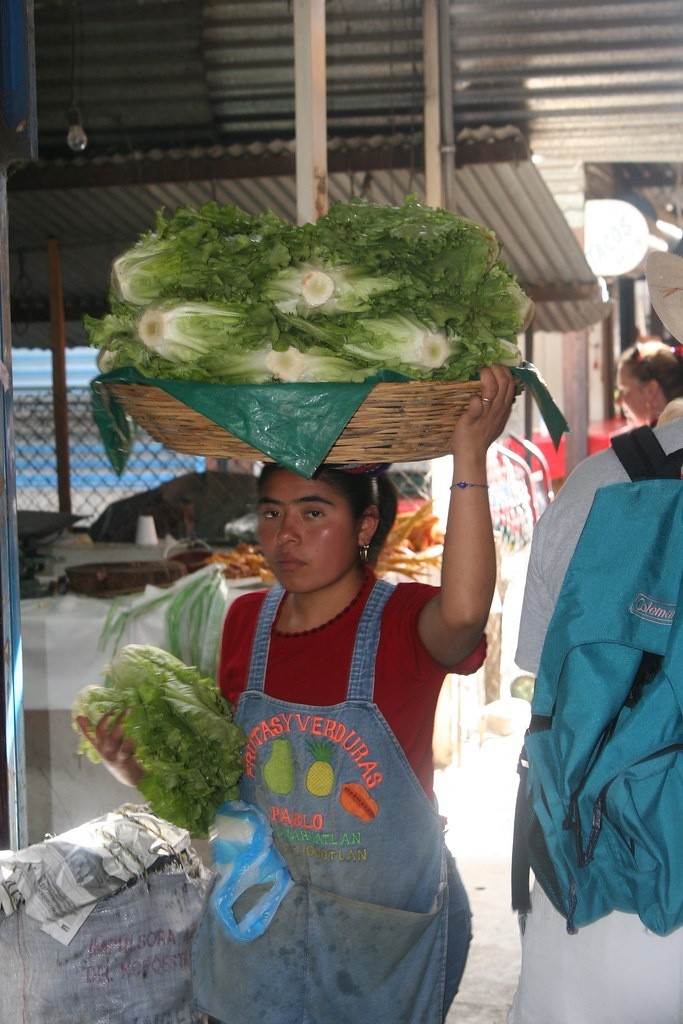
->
[482,397,494,403]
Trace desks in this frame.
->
[16,573,268,771]
[18,541,239,576]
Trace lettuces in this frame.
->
[82,193,536,386]
[70,643,244,840]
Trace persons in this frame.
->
[614,339,682,426]
[513,250,683,1023]
[76,361,537,1024]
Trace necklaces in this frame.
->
[271,573,370,638]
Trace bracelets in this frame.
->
[449,481,493,490]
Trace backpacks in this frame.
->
[507,425,683,936]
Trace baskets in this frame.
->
[93,354,524,464]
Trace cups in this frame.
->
[135,515,159,546]
